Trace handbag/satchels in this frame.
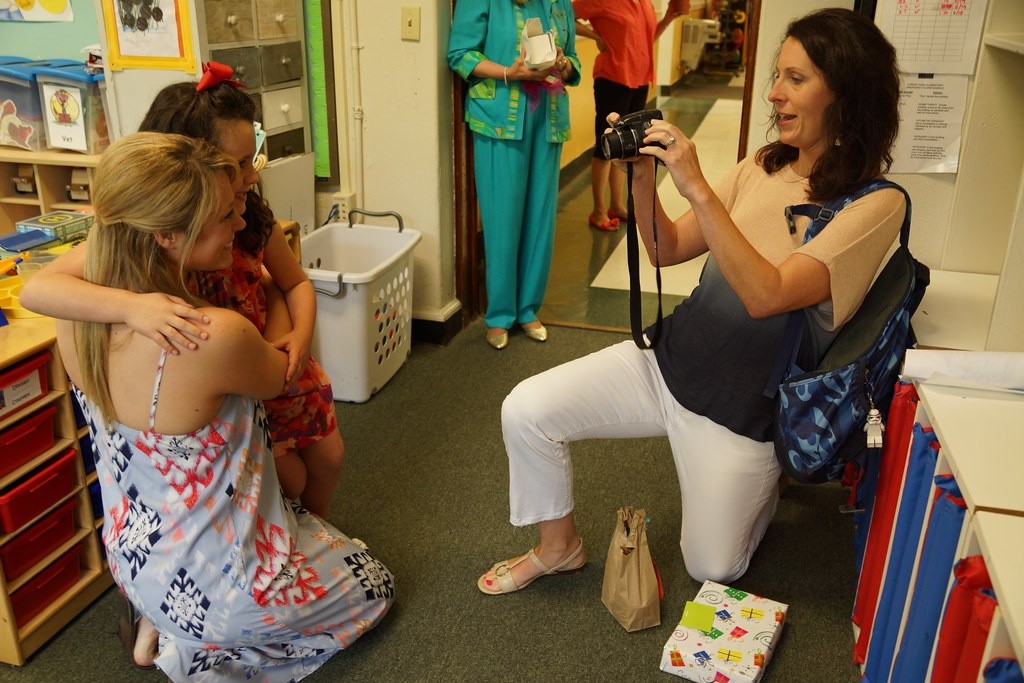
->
[764,179,930,483]
[601,506,664,632]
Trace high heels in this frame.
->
[486,329,508,349]
[521,320,547,341]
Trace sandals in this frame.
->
[478,538,586,595]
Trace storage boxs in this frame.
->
[0,351,108,630]
[0,54,116,155]
[659,580,789,683]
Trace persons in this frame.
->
[478,8,914,596]
[570,0,693,231]
[447,1,580,348]
[19,59,398,683]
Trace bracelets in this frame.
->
[504,66,508,86]
[560,57,568,72]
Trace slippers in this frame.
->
[128,602,155,670]
[608,208,637,223]
[589,216,619,231]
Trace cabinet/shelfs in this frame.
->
[0,147,115,250]
[95,0,316,236]
[908,1,1023,356]
[848,383,1023,683]
[0,305,122,668]
[922,511,1024,683]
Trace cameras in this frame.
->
[601,108,667,167]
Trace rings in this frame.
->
[557,61,562,67]
[665,136,675,146]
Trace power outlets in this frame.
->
[333,191,357,224]
